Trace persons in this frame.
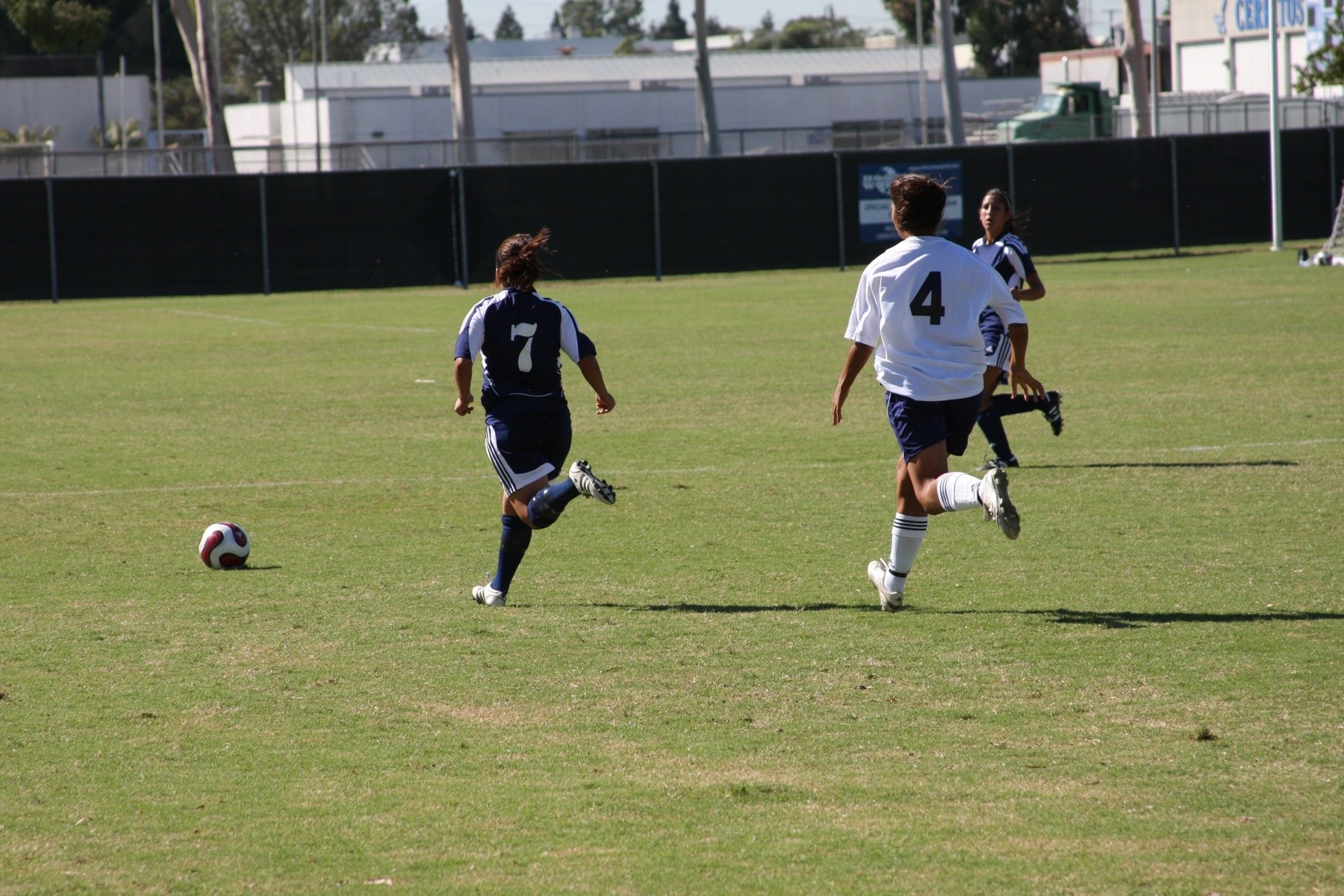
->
[450,226,618,606]
[830,173,1023,613]
[971,187,1060,471]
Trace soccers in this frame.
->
[198,521,250,571]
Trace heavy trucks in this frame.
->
[993,81,1335,144]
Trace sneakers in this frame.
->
[1042,390,1063,436]
[978,467,1020,540]
[868,558,904,612]
[979,454,1019,470]
[569,459,616,505]
[471,582,506,606]
